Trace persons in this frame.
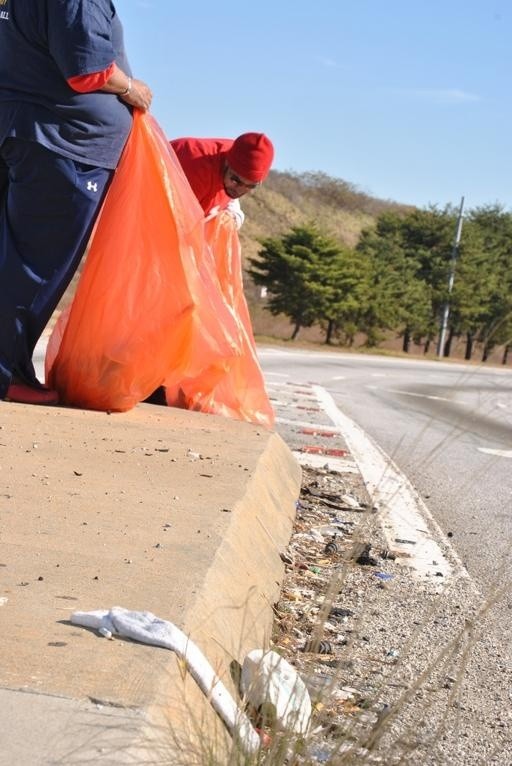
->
[0,0,154,408]
[169,132,275,231]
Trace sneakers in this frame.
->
[7,384,59,405]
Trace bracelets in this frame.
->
[118,77,132,97]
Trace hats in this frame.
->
[226,132,274,182]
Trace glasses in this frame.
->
[230,176,262,190]
